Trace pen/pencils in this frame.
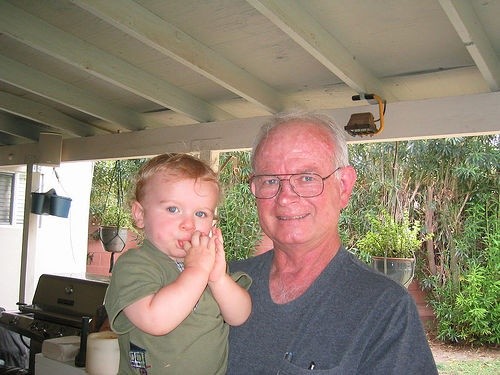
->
[276,351,292,375]
[307,361,315,370]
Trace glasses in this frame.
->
[249,167,341,199]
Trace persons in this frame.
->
[105,153,253,375]
[225,110,439,375]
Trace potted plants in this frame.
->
[100,204,128,252]
[356,210,434,289]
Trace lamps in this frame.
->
[343,94,386,138]
